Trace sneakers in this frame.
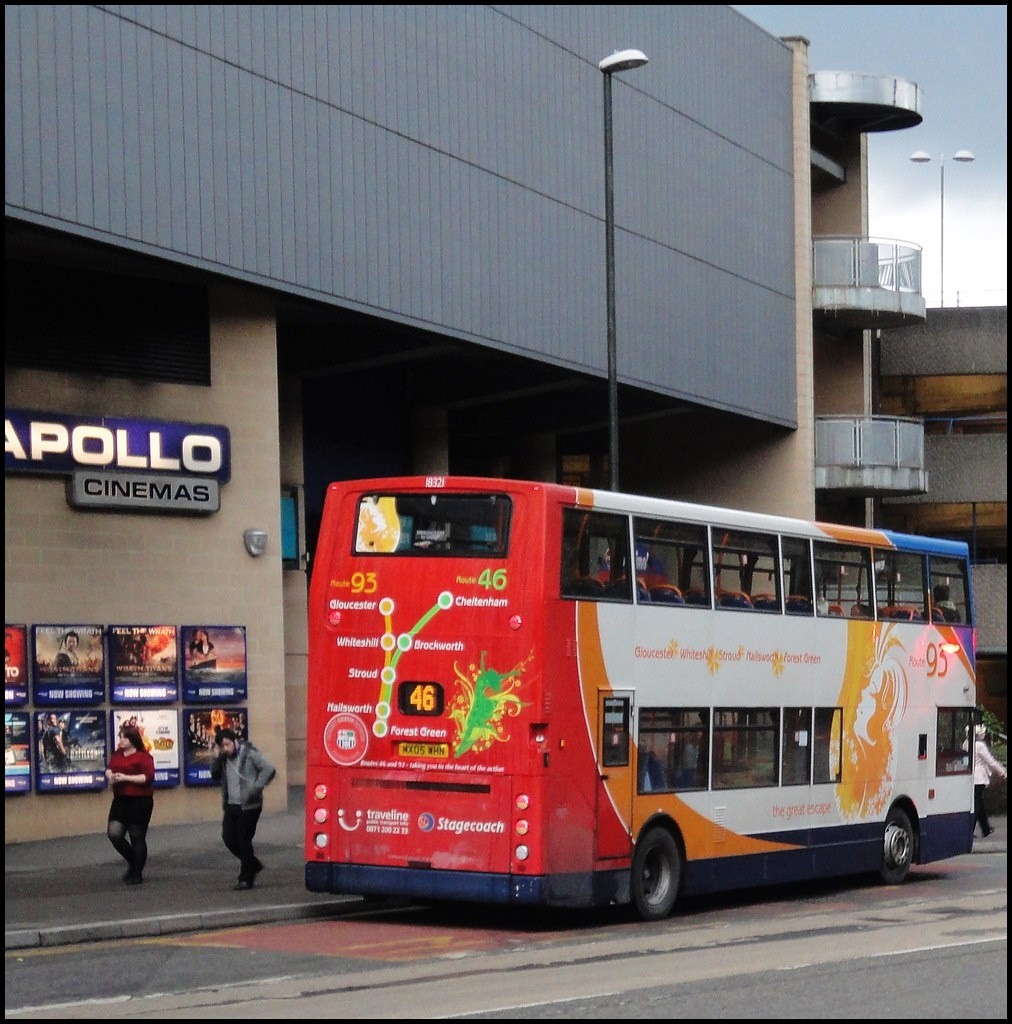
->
[232,857,266,890]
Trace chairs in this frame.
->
[603,751,692,792]
[411,535,945,625]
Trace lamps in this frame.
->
[243,527,267,557]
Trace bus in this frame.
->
[303,475,993,923]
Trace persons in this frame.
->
[932,584,961,622]
[189,713,246,759]
[211,729,276,890]
[964,724,1007,838]
[5,632,13,664]
[37,714,67,769]
[56,630,79,673]
[647,748,666,791]
[119,716,144,738]
[189,629,216,661]
[106,727,155,883]
[681,732,699,788]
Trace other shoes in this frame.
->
[981,827,995,836]
[122,869,145,885]
[973,833,977,840]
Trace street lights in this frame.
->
[599,47,649,493]
[910,149,975,308]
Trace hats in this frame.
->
[975,724,987,735]
